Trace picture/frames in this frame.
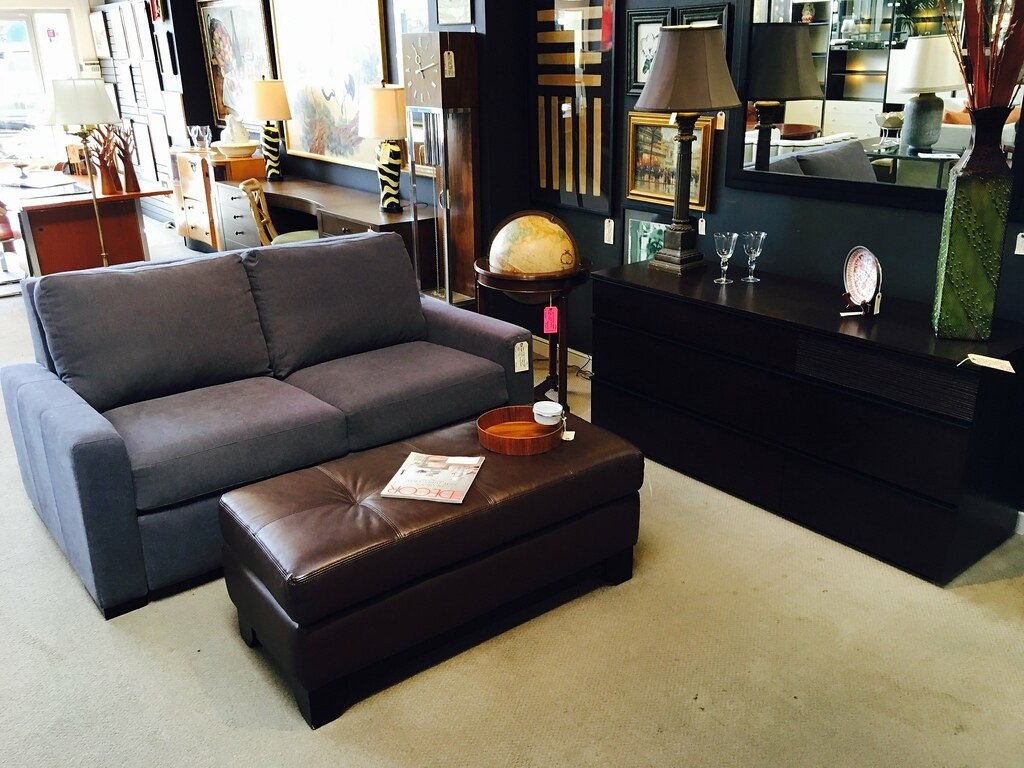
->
[87,11,111,60]
[626,10,673,98]
[264,0,388,175]
[623,209,697,264]
[624,112,715,214]
[677,2,732,70]
[191,0,273,146]
[435,0,476,25]
[400,106,443,179]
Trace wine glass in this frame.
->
[187,126,198,149]
[713,232,738,284]
[742,231,767,282]
[199,125,211,150]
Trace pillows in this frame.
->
[943,106,1021,125]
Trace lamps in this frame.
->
[374,85,408,216]
[898,33,968,158]
[740,20,823,172]
[51,75,125,268]
[634,23,744,277]
[253,78,292,183]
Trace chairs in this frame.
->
[239,177,320,246]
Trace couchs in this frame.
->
[931,97,1016,151]
[0,227,533,621]
[743,129,859,162]
[744,141,876,182]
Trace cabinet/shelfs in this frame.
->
[791,2,893,102]
[173,143,263,248]
[590,256,1024,590]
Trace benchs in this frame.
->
[220,401,647,733]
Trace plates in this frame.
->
[843,245,880,306]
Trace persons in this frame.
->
[641,164,700,188]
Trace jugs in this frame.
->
[220,115,249,141]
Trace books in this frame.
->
[381,451,485,504]
[918,153,960,159]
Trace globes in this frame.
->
[472,205,593,415]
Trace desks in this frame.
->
[0,169,174,315]
[773,123,822,138]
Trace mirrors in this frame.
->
[725,0,1024,213]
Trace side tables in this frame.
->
[864,147,963,188]
[861,138,900,155]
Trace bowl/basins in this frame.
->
[211,141,260,157]
[532,402,562,425]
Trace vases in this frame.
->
[930,103,1014,343]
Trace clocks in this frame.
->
[400,32,443,113]
